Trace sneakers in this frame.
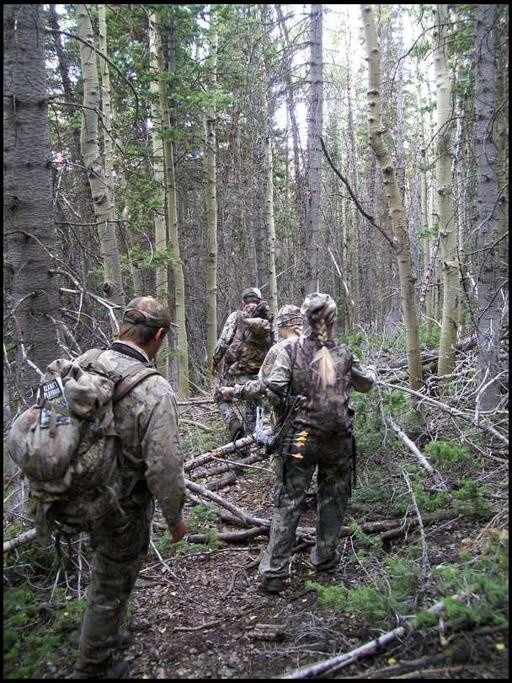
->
[314,549,340,571]
[77,634,133,679]
[261,577,284,592]
[228,418,244,442]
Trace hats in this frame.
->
[277,304,303,326]
[122,294,183,331]
[242,286,263,299]
[301,292,338,326]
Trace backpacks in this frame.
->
[7,349,155,533]
[226,306,274,365]
[292,344,355,434]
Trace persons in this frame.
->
[37,292,192,679]
[257,290,377,594]
[210,286,274,459]
[216,303,317,511]
